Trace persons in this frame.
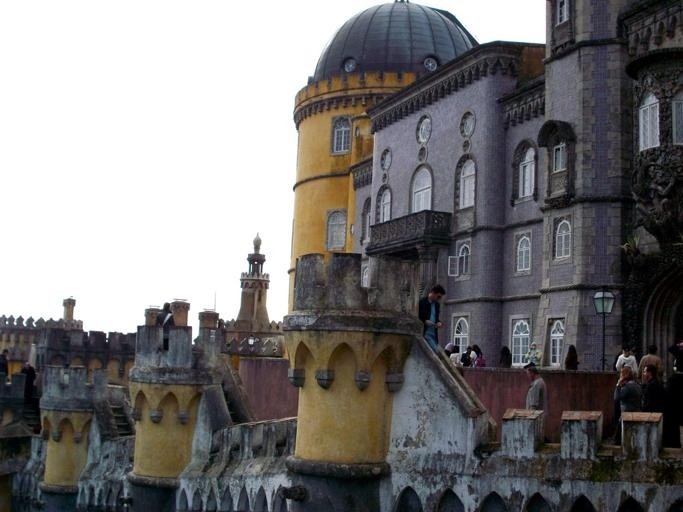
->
[419,284,447,345]
[443,343,486,368]
[0,349,8,377]
[524,361,548,416]
[499,346,512,368]
[612,339,683,448]
[564,344,580,370]
[20,362,36,404]
[525,342,544,370]
[155,303,176,350]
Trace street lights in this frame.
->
[590,287,616,371]
